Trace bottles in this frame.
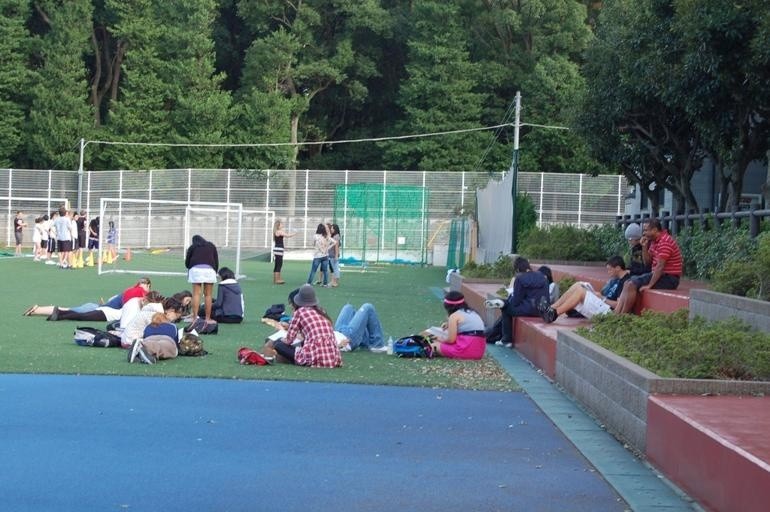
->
[387,337,394,355]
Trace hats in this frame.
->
[624,222,643,241]
[177,334,209,357]
[294,283,320,307]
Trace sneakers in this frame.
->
[484,298,506,310]
[128,338,155,365]
[495,339,513,348]
[22,304,61,323]
[538,301,558,324]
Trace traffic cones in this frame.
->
[69,246,119,269]
[123,246,132,262]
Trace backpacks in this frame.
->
[72,325,122,349]
[391,334,437,360]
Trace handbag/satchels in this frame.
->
[184,317,220,336]
